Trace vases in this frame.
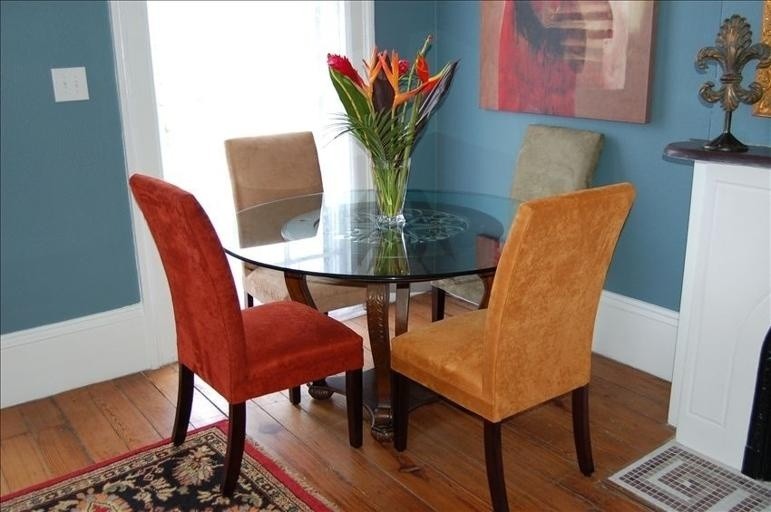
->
[369,159,411,230]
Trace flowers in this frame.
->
[326,34,463,216]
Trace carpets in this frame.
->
[607,439,771,511]
[1,419,338,512]
